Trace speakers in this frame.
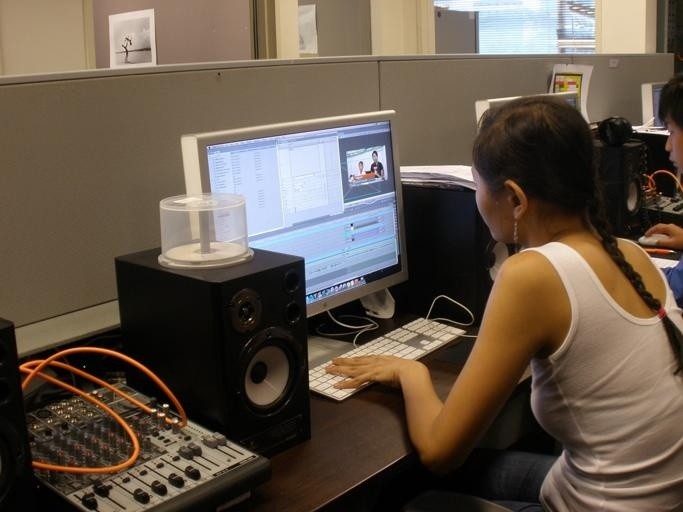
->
[390,185,516,320]
[592,139,648,237]
[636,130,678,198]
[113,247,311,457]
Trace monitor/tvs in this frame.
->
[641,82,665,129]
[181,109,410,319]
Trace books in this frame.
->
[640,81,668,127]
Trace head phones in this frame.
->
[598,117,632,146]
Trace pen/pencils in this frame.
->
[643,248,677,255]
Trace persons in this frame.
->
[644,75,683,297]
[355,161,366,176]
[325,96,683,512]
[371,150,384,178]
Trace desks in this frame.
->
[42,313,535,512]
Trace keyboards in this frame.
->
[308,317,467,401]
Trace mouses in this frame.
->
[638,234,668,247]
